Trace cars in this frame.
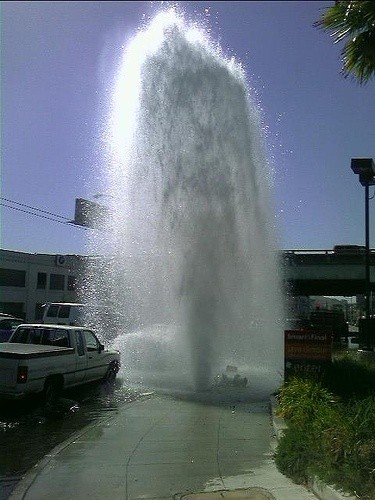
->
[0,317,29,342]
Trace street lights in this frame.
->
[350,158,375,348]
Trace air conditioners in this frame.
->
[56,255,67,267]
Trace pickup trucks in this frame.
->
[0,323,121,400]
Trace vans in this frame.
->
[334,245,368,255]
[42,302,84,326]
